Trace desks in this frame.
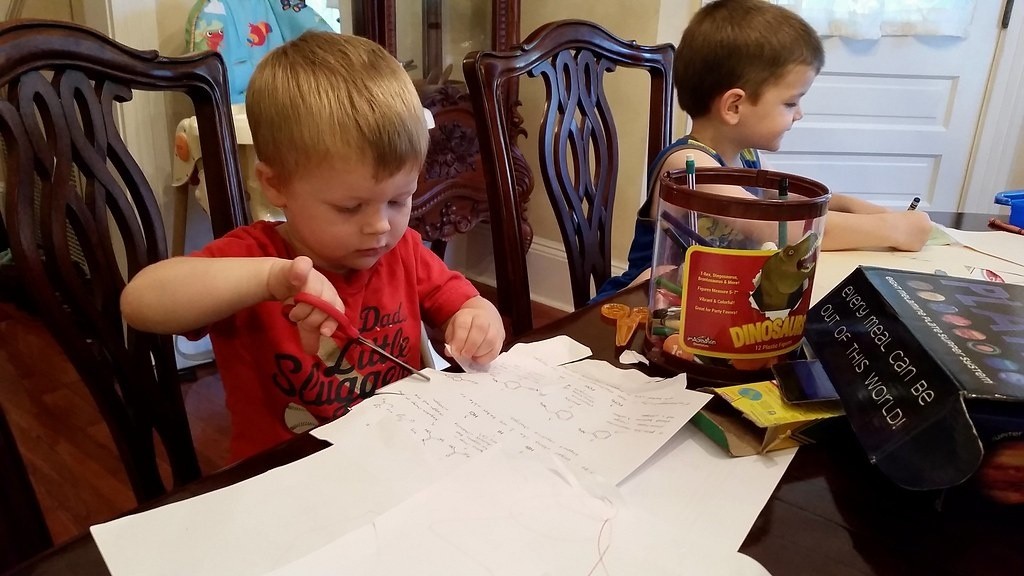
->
[168,104,256,264]
[34,213,1024,576]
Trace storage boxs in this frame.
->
[803,265,1024,534]
[689,376,844,458]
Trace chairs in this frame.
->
[0,16,248,576]
[462,19,675,338]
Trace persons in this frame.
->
[591,1,932,308]
[120,29,505,476]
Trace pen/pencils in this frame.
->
[987,217,1024,235]
[650,153,790,336]
[907,197,921,212]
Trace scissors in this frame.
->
[281,292,430,381]
[601,302,649,347]
